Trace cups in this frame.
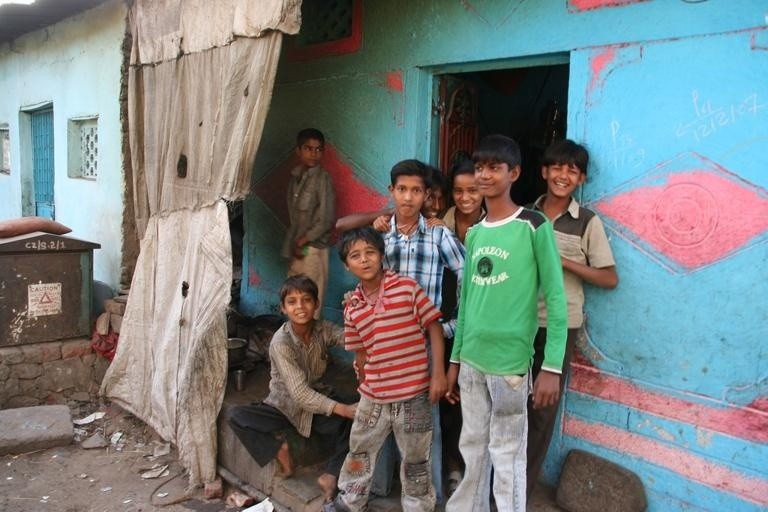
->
[232,369,247,391]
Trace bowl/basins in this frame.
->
[227,338,247,363]
